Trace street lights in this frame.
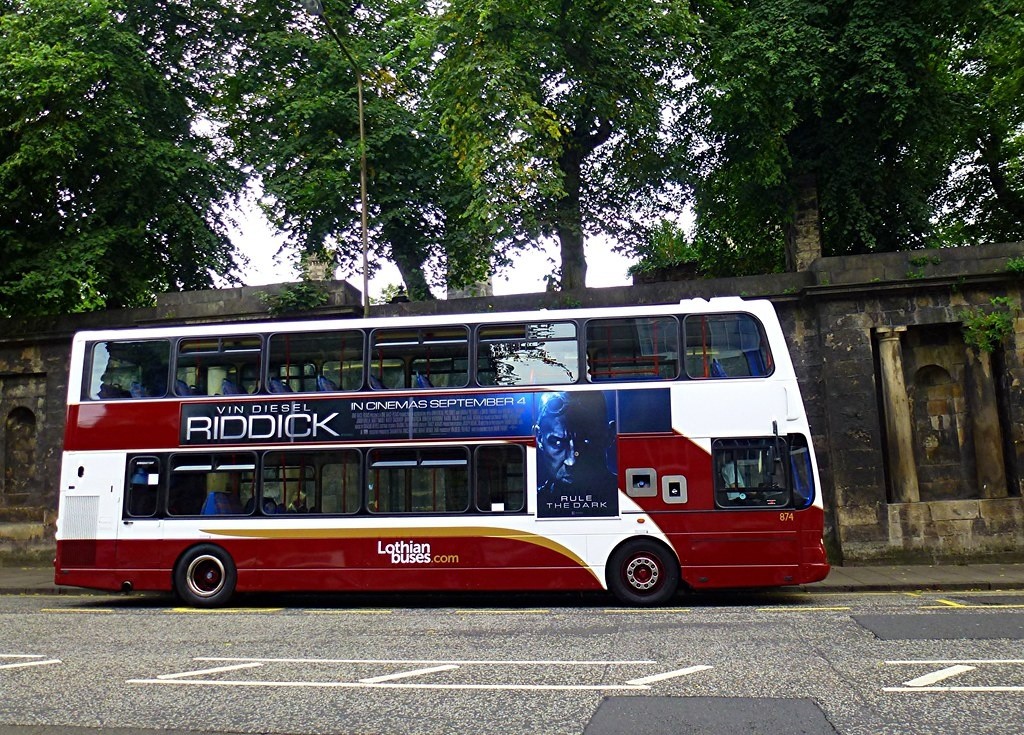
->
[288,0,370,317]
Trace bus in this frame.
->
[54,296,829,606]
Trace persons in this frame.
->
[721,450,763,504]
[531,392,618,519]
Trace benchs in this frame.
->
[197,490,323,515]
[87,356,730,401]
